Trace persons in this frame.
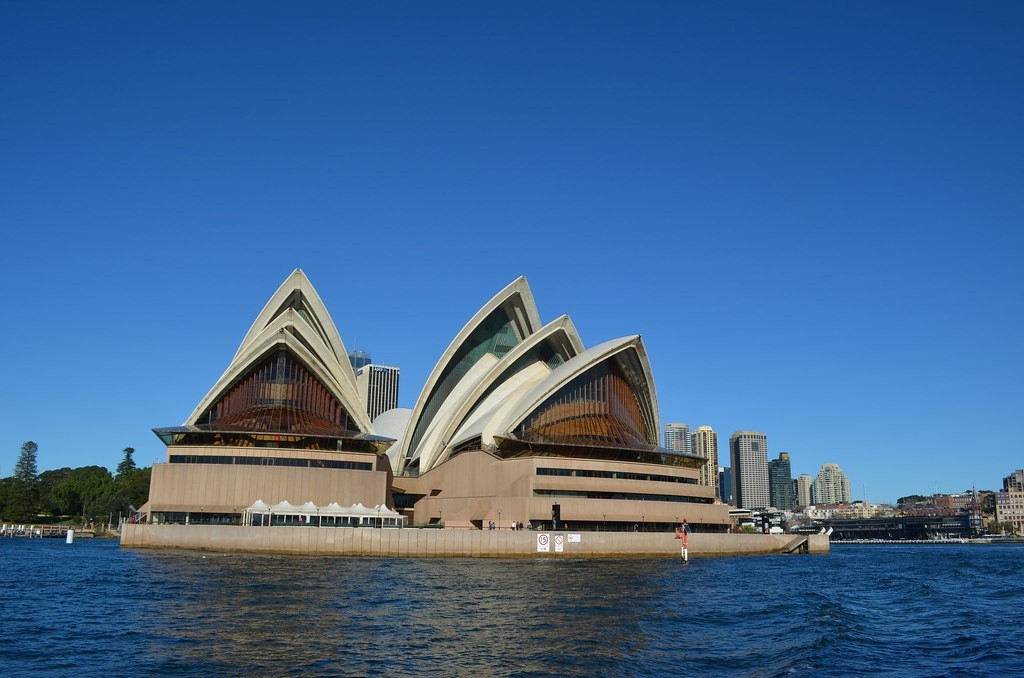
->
[527,521,542,531]
[512,521,523,531]
[489,521,495,530]
[565,524,567,528]
[738,526,742,533]
[299,518,302,525]
[727,527,730,533]
[634,524,638,532]
[131,516,135,524]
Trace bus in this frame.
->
[982,535,1007,541]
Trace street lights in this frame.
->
[439,508,442,528]
[498,508,501,529]
[316,506,320,527]
[642,513,645,531]
[676,515,679,528]
[722,516,725,532]
[377,506,381,527]
[232,506,237,525]
[200,506,204,524]
[700,516,703,532]
[603,512,606,531]
[267,505,271,526]
